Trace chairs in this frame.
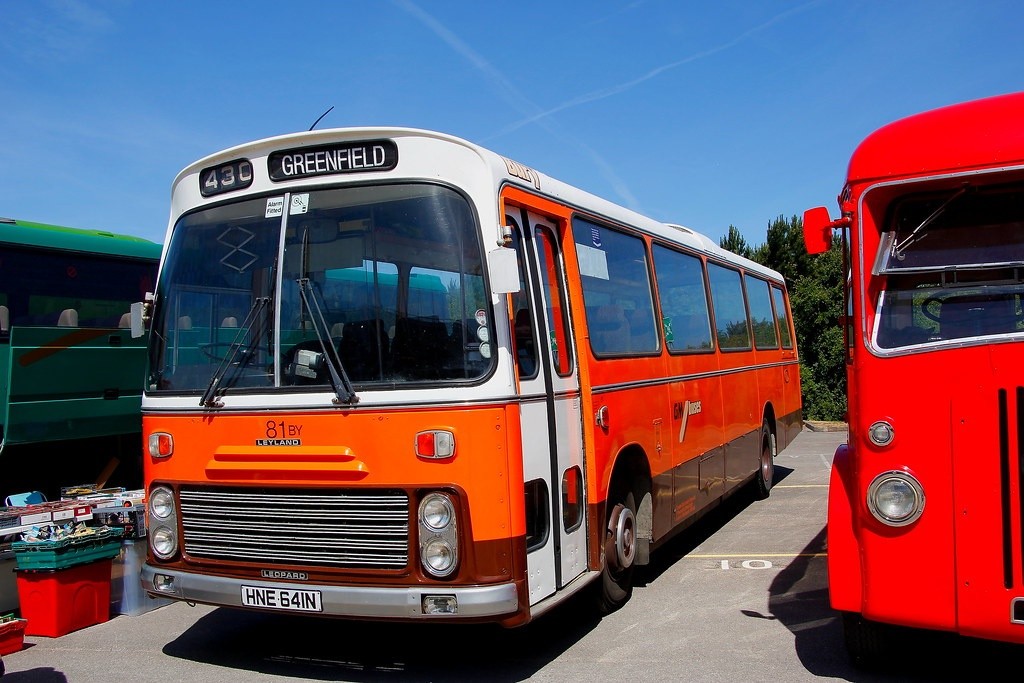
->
[5,490,48,507]
[630,308,655,352]
[337,319,389,382]
[592,304,631,352]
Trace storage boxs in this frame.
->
[13,555,116,637]
[92,505,146,539]
[0,618,28,656]
[67,506,91,516]
[19,510,53,526]
[111,540,178,617]
[52,509,74,522]
[11,527,124,569]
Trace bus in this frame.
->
[799,90,1024,645]
[0,214,455,506]
[129,126,803,633]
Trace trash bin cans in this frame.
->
[13,526,125,638]
